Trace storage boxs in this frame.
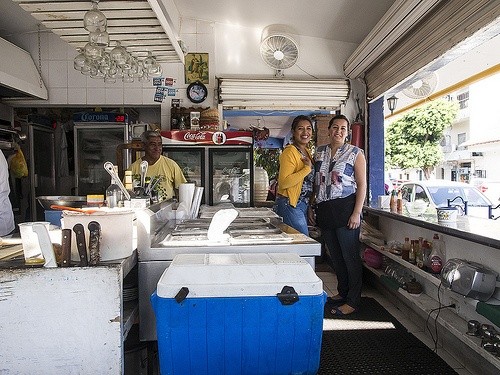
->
[151,253,328,375]
[45,207,139,262]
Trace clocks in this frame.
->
[187,80,208,104]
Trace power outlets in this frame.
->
[449,297,459,313]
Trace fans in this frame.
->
[260,33,300,78]
[400,66,437,99]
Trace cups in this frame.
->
[87,195,105,207]
[18,221,50,265]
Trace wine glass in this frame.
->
[73,0,163,83]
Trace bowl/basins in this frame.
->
[36,195,87,208]
[388,241,403,255]
[436,207,458,222]
[405,202,428,215]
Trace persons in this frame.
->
[308,114,368,318]
[0,149,16,238]
[272,115,316,238]
[123,131,187,205]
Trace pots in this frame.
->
[60,206,137,262]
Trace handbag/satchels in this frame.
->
[7,143,28,177]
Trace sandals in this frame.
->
[330,306,356,316]
[324,295,345,303]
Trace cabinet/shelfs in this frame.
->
[359,202,500,370]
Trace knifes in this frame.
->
[33,221,101,268]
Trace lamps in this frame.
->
[387,94,399,115]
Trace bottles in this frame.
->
[402,234,443,273]
[397,190,403,213]
[253,165,270,202]
[177,118,187,130]
[106,165,123,207]
[125,171,157,205]
[390,190,397,212]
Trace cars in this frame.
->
[384,178,500,222]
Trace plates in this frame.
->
[123,282,138,302]
